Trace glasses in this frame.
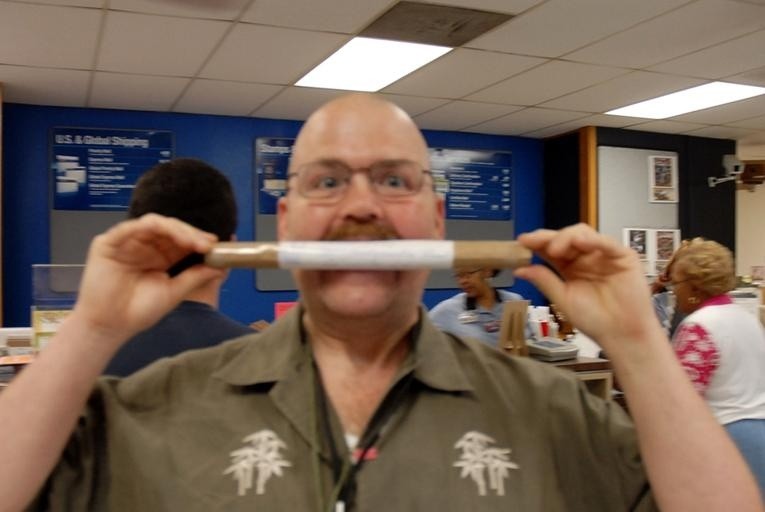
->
[287,160,434,201]
[666,279,686,291]
[453,266,483,278]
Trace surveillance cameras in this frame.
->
[708,153,745,188]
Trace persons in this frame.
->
[668,237,765,497]
[98,158,262,378]
[425,258,526,348]
[0,92,765,511]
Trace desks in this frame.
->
[574,370,614,402]
